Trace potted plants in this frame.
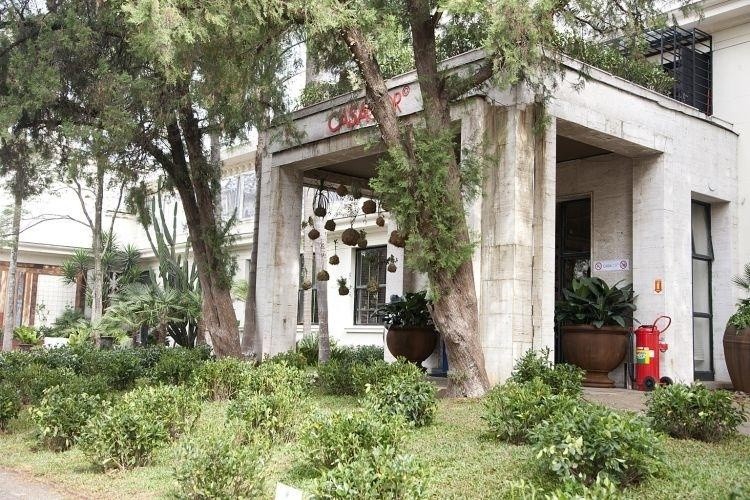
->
[13,327,38,354]
[370,288,440,372]
[554,276,641,387]
[723,265,750,393]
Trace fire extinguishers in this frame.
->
[628,316,673,391]
